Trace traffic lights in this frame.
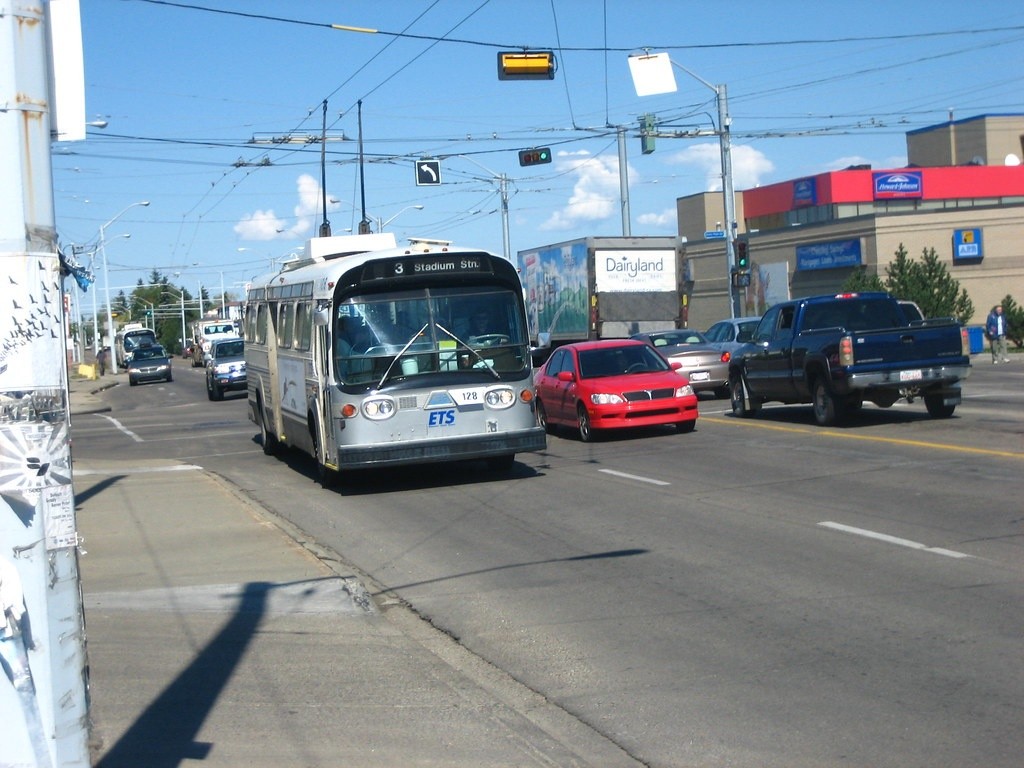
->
[146,310,151,316]
[330,198,424,234]
[734,238,749,272]
[735,274,750,288]
[518,147,552,167]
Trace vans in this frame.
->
[896,299,925,325]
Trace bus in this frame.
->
[242,233,551,481]
[117,322,156,366]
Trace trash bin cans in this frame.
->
[967,327,984,354]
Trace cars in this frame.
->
[125,342,173,386]
[629,329,732,399]
[184,346,193,358]
[532,338,700,442]
[703,318,765,365]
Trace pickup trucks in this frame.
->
[729,290,973,426]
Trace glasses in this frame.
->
[476,316,488,320]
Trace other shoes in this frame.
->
[1002,359,1010,363]
[993,361,998,364]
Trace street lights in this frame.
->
[89,233,132,356]
[176,271,204,320]
[100,202,151,377]
[161,291,186,349]
[193,261,226,320]
[237,247,274,273]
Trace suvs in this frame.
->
[204,338,246,402]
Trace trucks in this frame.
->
[516,236,695,366]
[191,321,239,348]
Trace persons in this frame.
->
[95,347,107,377]
[986,305,1013,364]
